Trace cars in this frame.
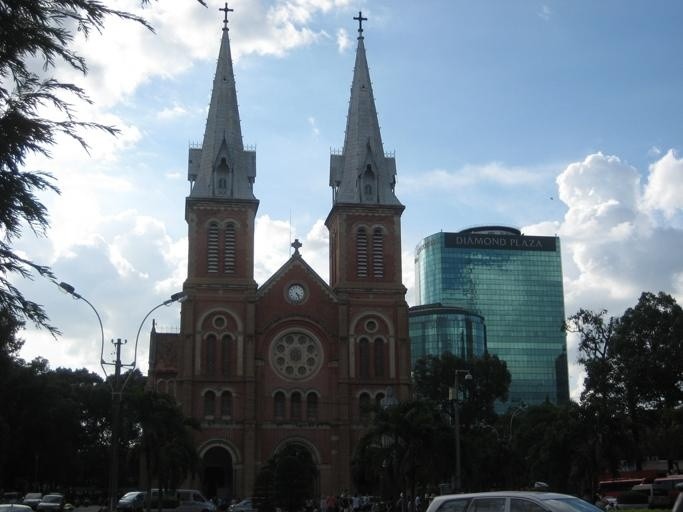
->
[0,492,74,512]
[116,490,146,511]
[422,491,605,512]
[228,497,252,511]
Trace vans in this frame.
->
[632,484,672,508]
[147,487,217,510]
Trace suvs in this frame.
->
[595,495,623,512]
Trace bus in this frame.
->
[596,472,683,506]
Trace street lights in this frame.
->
[381,449,407,512]
[49,278,189,512]
[448,369,474,492]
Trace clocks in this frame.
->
[288,285,305,302]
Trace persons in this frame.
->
[1,478,529,512]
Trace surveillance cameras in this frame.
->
[464,373,473,382]
